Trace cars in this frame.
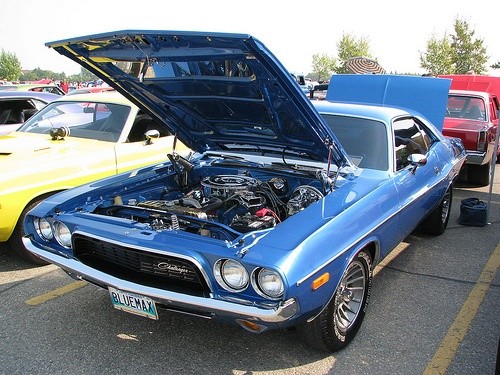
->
[0,88,195,267]
[22,30,469,354]
[0,84,67,96]
[437,74,500,186]
[0,91,113,140]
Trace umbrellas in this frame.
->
[347,56,386,74]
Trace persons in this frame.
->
[4,108,25,125]
[308,80,315,99]
[59,78,109,94]
[0,76,11,86]
